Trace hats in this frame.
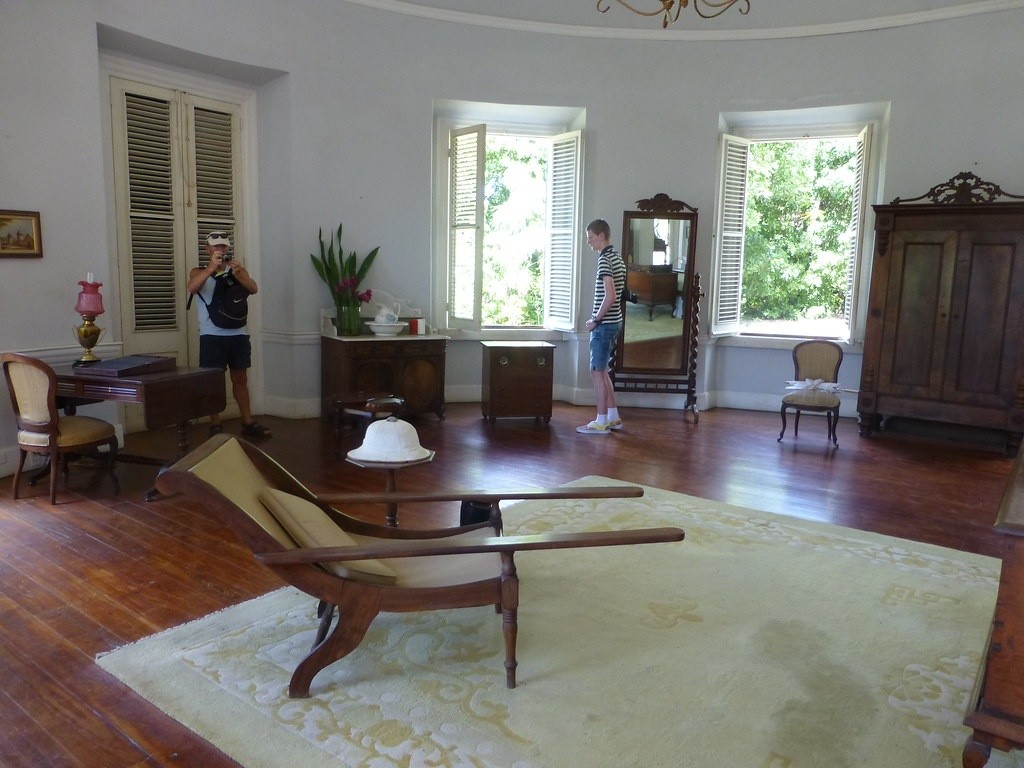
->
[346,416,430,462]
[207,232,230,246]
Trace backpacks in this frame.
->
[194,266,248,329]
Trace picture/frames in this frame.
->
[0,209,41,258]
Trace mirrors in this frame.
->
[609,193,704,426]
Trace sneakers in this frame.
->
[607,418,624,430]
[577,421,610,434]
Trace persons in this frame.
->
[576,218,627,433]
[188,232,272,439]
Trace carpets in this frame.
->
[77,475,1024,768]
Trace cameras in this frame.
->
[218,253,232,262]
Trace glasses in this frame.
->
[206,233,228,240]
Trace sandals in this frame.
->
[208,423,223,438]
[241,421,271,438]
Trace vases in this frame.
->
[336,306,361,335]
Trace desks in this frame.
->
[629,270,678,320]
[345,450,436,526]
[51,366,227,502]
[327,391,407,455]
[320,333,451,425]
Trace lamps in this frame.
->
[73,281,104,362]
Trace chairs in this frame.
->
[1,353,120,504]
[144,435,685,700]
[778,341,845,448]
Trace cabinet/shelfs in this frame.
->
[856,171,1024,440]
[481,341,557,424]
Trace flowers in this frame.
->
[311,223,380,305]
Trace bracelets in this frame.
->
[593,316,600,322]
